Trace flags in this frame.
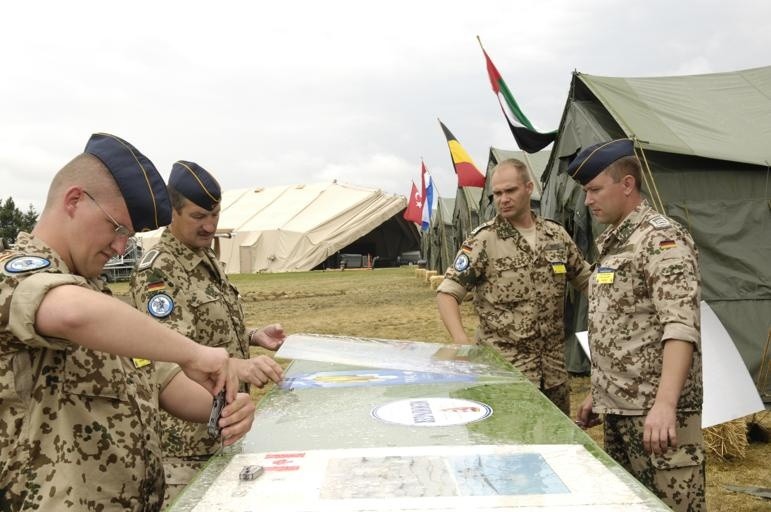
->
[440,122,486,189]
[485,53,560,153]
[420,165,438,229]
[404,184,422,223]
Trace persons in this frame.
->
[435,159,590,418]
[133,156,287,500]
[0,131,254,512]
[563,138,706,511]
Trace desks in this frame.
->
[161,345,675,512]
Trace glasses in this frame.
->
[84,191,130,238]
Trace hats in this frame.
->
[567,138,634,185]
[85,132,173,232]
[170,160,222,210]
